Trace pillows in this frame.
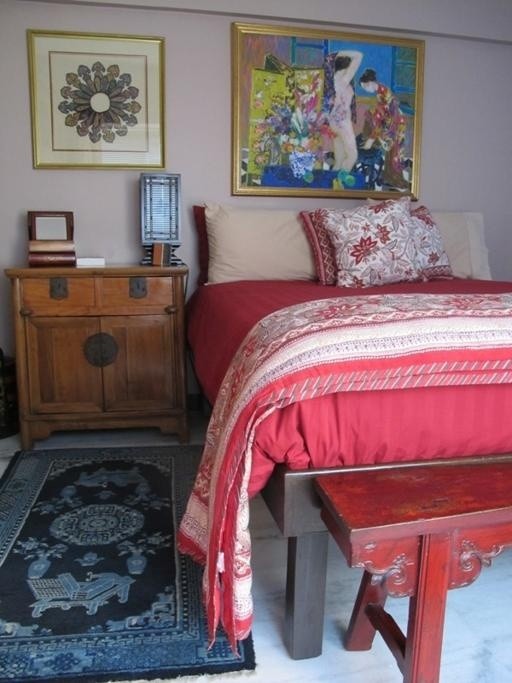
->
[316,194,414,286]
[301,211,339,285]
[204,201,318,283]
[422,212,489,279]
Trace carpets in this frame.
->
[0,444,258,683]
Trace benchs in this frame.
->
[315,464,511,678]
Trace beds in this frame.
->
[185,209,512,658]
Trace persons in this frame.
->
[327,50,363,191]
[359,68,411,192]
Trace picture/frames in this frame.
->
[228,21,426,200]
[26,30,167,174]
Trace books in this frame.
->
[28,239,77,266]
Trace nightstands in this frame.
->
[6,265,190,454]
